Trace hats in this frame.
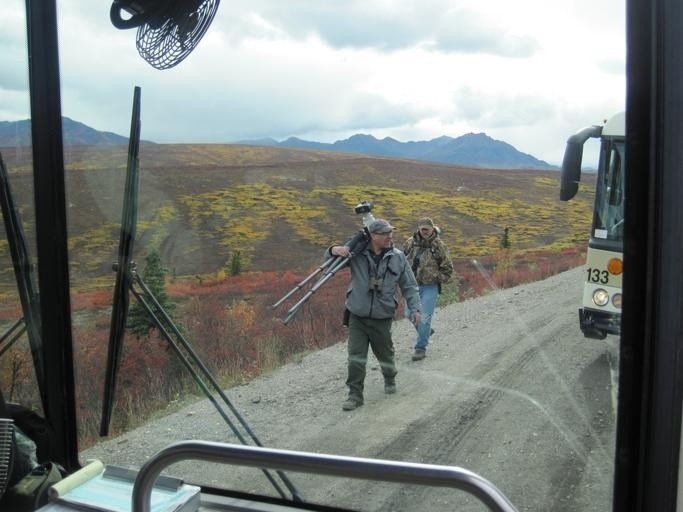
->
[417,217,433,229]
[369,220,397,233]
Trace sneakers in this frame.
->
[384,375,395,394]
[411,350,425,361]
[341,397,362,410]
[431,328,434,335]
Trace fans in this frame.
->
[109,0,221,70]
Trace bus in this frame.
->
[559,111,626,342]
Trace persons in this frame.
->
[321,218,424,410]
[399,214,456,363]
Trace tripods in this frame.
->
[271,226,372,326]
